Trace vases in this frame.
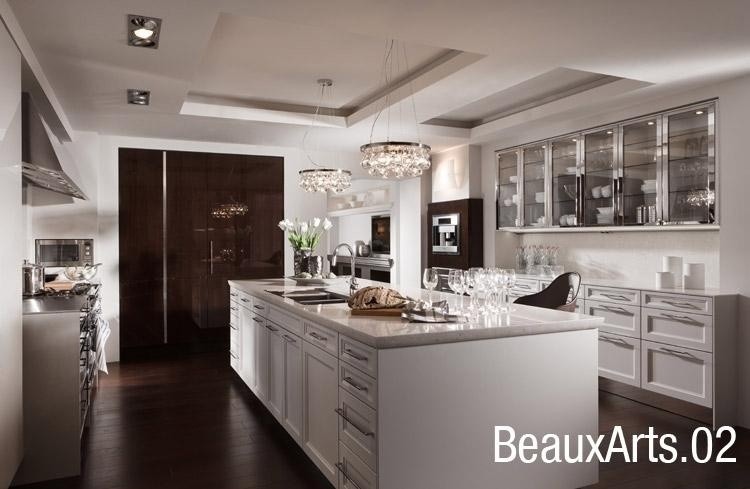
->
[294,249,312,275]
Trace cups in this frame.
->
[535,150,544,179]
[559,214,575,225]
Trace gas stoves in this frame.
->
[34,284,94,296]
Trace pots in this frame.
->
[22,260,45,296]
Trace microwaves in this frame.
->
[34,238,94,266]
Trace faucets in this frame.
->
[332,242,358,296]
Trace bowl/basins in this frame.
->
[45,274,58,282]
[63,266,97,283]
[509,176,517,183]
[596,207,612,213]
[336,203,350,210]
[349,201,364,208]
[591,184,611,198]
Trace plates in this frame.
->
[596,214,612,224]
[504,194,517,206]
[535,192,544,203]
[287,276,347,285]
[640,180,656,193]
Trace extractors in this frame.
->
[22,91,89,201]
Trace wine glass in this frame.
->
[423,267,515,322]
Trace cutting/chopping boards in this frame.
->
[352,307,405,319]
[45,282,76,290]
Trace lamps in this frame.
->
[360,39,432,181]
[298,78,352,193]
[209,200,249,219]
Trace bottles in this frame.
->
[685,133,708,156]
[359,245,370,257]
[515,245,559,274]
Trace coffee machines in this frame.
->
[430,212,461,256]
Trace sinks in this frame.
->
[283,292,348,305]
[265,287,329,295]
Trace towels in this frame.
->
[401,296,459,323]
[95,315,111,375]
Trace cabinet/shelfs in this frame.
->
[494,97,721,233]
[230,282,376,489]
[501,272,715,432]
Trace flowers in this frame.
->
[277,216,333,258]
